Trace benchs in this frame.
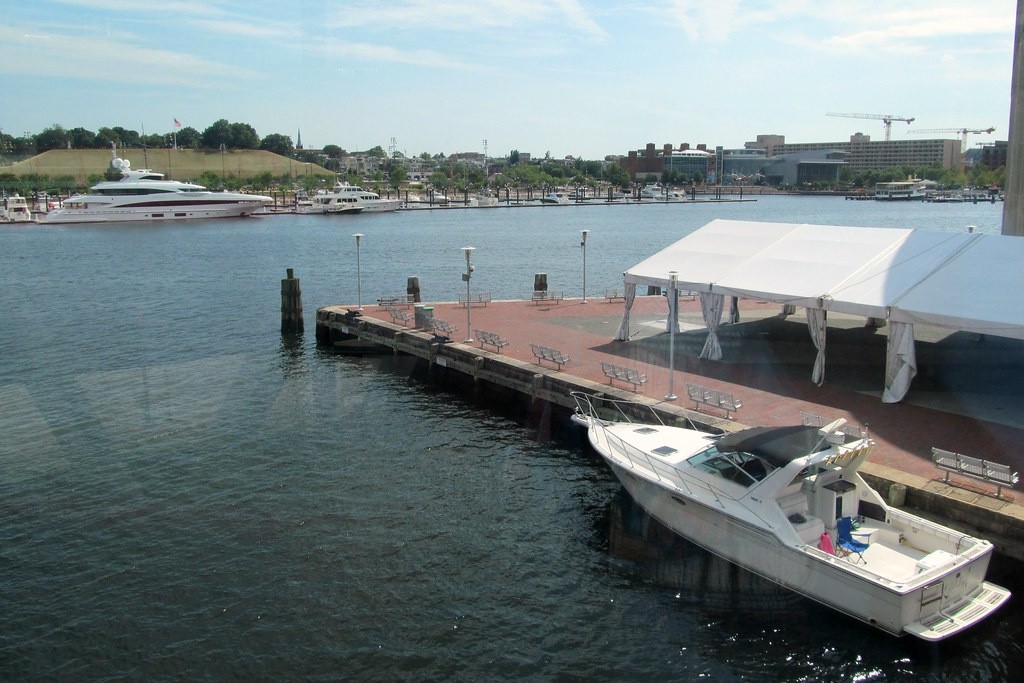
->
[600,361,649,392]
[932,446,1019,498]
[530,343,572,371]
[426,317,458,339]
[606,286,626,303]
[851,515,904,547]
[532,290,563,305]
[458,292,491,308]
[686,383,742,420]
[474,328,510,353]
[679,290,700,300]
[377,293,415,326]
[799,410,868,438]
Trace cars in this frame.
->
[619,189,630,194]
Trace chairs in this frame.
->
[835,516,871,564]
[777,491,826,545]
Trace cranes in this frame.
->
[975,142,995,148]
[825,111,915,141]
[907,128,996,172]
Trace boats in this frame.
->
[328,203,364,214]
[46,143,273,222]
[570,391,1012,641]
[302,186,404,212]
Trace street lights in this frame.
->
[497,186,555,205]
[388,190,389,199]
[4,194,7,210]
[460,246,476,342]
[576,185,642,202]
[430,188,432,207]
[269,189,297,209]
[666,185,742,201]
[445,188,447,204]
[378,188,380,197]
[32,192,34,210]
[58,194,61,208]
[46,194,48,212]
[580,230,590,304]
[220,143,226,185]
[397,187,399,199]
[405,188,408,208]
[352,234,364,311]
[465,188,466,206]
